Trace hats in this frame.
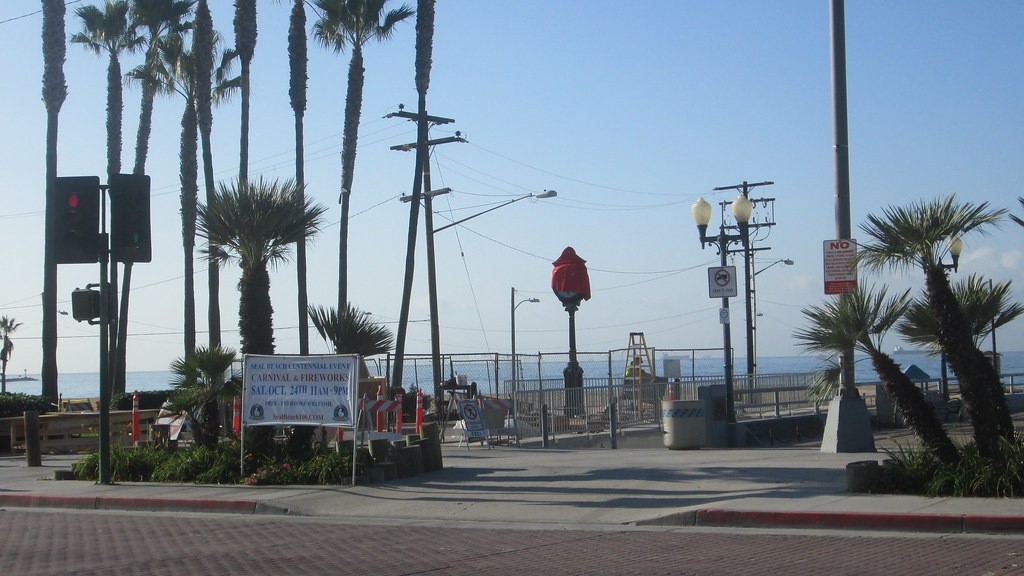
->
[635,357,643,365]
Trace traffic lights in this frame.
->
[72,289,100,319]
[110,173,151,263]
[53,176,99,264]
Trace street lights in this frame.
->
[424,190,557,424]
[691,193,753,449]
[509,286,540,421]
[743,258,794,407]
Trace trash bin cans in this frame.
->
[698,384,728,448]
